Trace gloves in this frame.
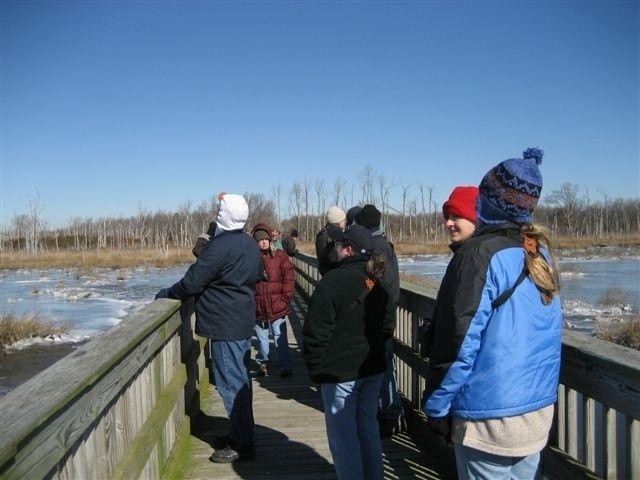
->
[428,415,450,446]
[154,287,167,299]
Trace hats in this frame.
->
[253,230,270,242]
[327,205,381,254]
[291,229,297,237]
[443,186,480,224]
[477,148,543,224]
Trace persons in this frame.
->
[157,194,261,464]
[300,204,401,479]
[421,146,559,479]
[250,223,300,378]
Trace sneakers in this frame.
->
[280,369,292,377]
[210,435,255,462]
[259,361,271,377]
